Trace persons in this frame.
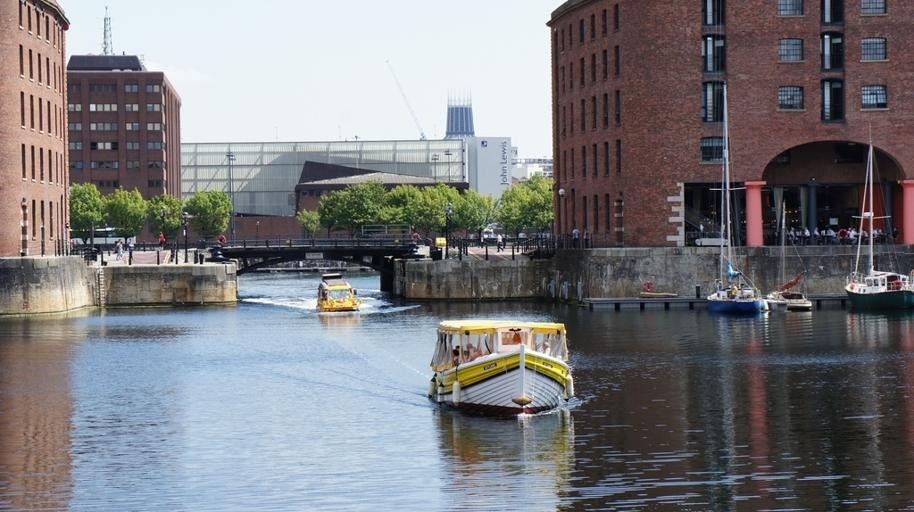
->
[158,232,166,251]
[572,226,581,248]
[732,284,738,298]
[114,238,134,261]
[582,224,590,248]
[454,343,477,364]
[788,225,899,245]
[497,233,504,252]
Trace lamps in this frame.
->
[558,188,570,204]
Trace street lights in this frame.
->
[432,154,439,188]
[180,211,190,263]
[442,200,453,258]
[444,149,453,188]
[254,219,259,246]
[230,158,237,246]
[159,204,166,250]
[226,153,234,201]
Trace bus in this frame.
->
[69,225,136,253]
[483,221,549,245]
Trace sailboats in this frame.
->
[703,76,770,313]
[840,116,914,313]
[764,200,815,313]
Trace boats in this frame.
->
[315,313,362,327]
[426,316,573,420]
[640,290,681,298]
[317,273,362,313]
[433,404,575,499]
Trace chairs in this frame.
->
[772,221,899,248]
